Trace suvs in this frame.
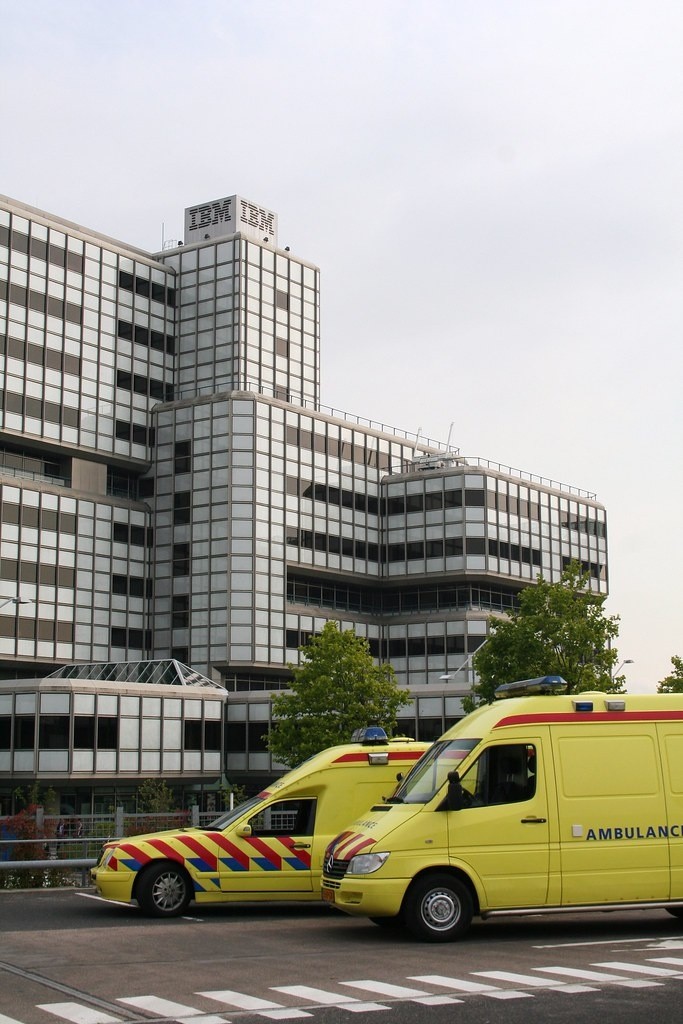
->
[90,726,534,921]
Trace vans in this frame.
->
[316,676,683,944]
